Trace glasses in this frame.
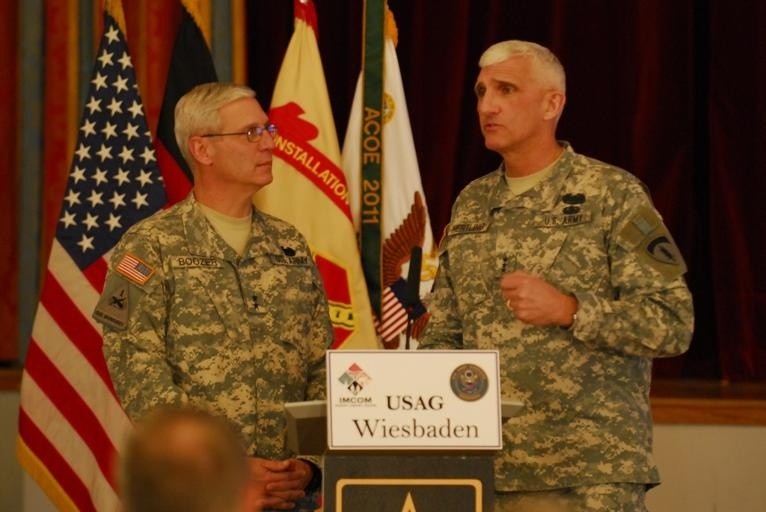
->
[199,123,278,143]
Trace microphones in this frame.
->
[397,244,425,349]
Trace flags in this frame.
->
[252,0,389,352]
[374,278,427,344]
[18,0,170,512]
[336,0,446,351]
[151,0,220,214]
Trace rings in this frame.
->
[505,298,515,312]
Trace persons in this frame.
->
[92,80,338,512]
[413,36,698,512]
[111,398,268,512]
